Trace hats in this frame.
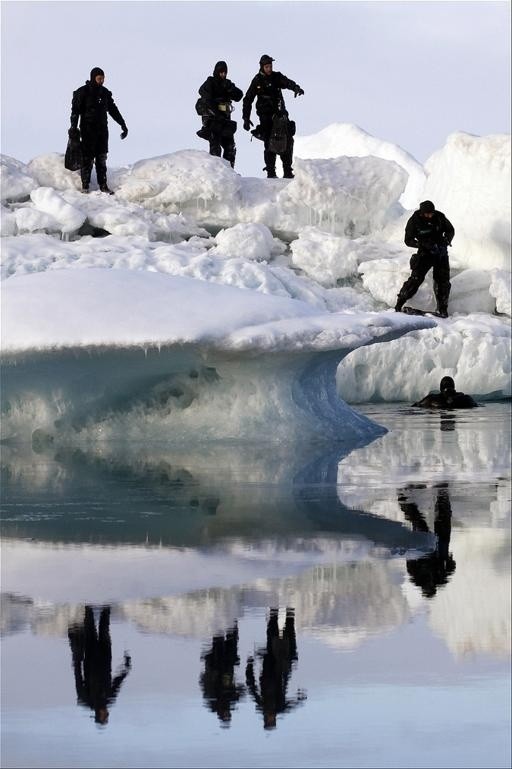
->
[420,201,435,220]
[260,55,272,75]
[213,61,227,79]
[90,67,104,87]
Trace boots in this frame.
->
[266,167,278,178]
[100,184,115,195]
[439,306,448,318]
[395,294,406,312]
[283,169,295,178]
[82,183,89,192]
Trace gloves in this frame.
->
[244,117,253,131]
[120,125,128,139]
[293,85,304,98]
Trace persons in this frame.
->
[408,405,464,434]
[394,482,458,600]
[242,54,307,178]
[194,58,244,168]
[198,618,246,726]
[243,607,309,731]
[68,65,128,195]
[66,603,135,727]
[410,376,482,412]
[395,200,457,319]
